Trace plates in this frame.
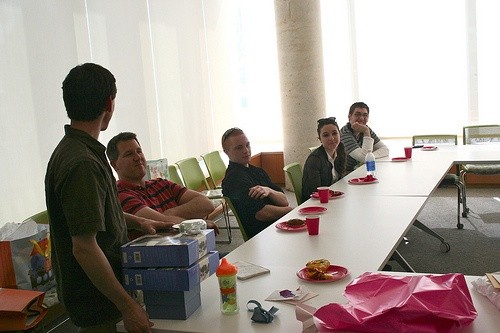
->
[392,157,410,160]
[309,190,345,199]
[275,220,307,231]
[299,206,327,214]
[296,264,351,283]
[349,177,378,184]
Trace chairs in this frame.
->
[282,162,302,205]
[169,150,240,243]
[412,134,464,231]
[463,125,500,218]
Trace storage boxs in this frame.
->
[120,229,219,320]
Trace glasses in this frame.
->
[352,112,368,118]
[223,127,242,143]
[317,117,336,126]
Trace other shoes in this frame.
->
[382,264,392,271]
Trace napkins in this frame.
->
[233,260,270,280]
[264,285,319,304]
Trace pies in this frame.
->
[306,259,334,280]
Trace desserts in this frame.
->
[286,219,304,226]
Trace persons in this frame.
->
[45,63,154,333]
[221,127,293,238]
[302,116,347,203]
[340,102,389,179]
[106,132,220,236]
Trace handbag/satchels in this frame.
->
[143,158,168,181]
[0,219,56,295]
[0,288,50,331]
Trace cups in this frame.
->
[305,216,319,235]
[404,147,413,158]
[317,187,330,203]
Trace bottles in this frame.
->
[216,257,239,315]
[365,149,375,177]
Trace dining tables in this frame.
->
[116,196,500,333]
[330,145,500,253]
